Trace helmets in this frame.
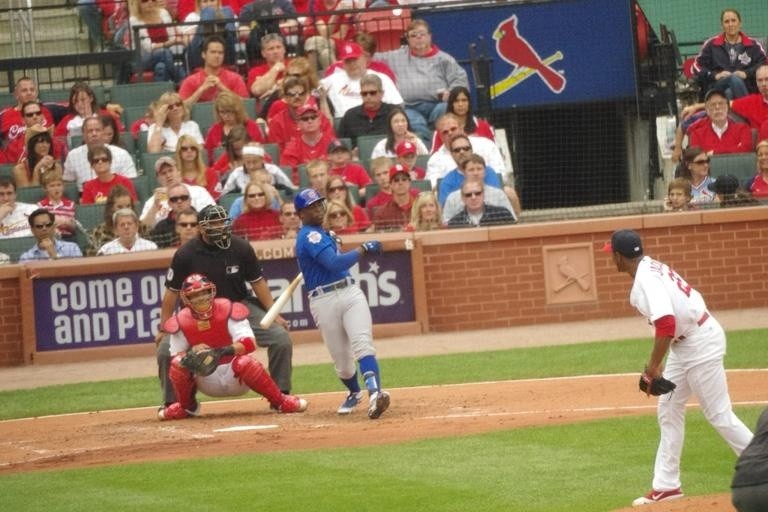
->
[197,204,233,250]
[180,273,216,318]
[294,188,326,211]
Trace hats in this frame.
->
[388,163,409,178]
[339,43,362,60]
[704,88,726,100]
[297,102,316,115]
[602,229,643,259]
[327,139,348,153]
[303,35,327,53]
[707,172,740,194]
[154,155,176,173]
[395,141,417,157]
[24,124,54,155]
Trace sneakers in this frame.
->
[277,394,307,412]
[336,390,366,415]
[367,391,391,418]
[162,398,203,420]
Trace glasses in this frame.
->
[464,191,483,198]
[442,125,458,135]
[90,157,111,163]
[359,90,378,96]
[180,146,197,152]
[688,158,711,165]
[26,109,43,118]
[179,222,198,228]
[167,102,183,110]
[410,30,426,38]
[451,145,471,152]
[394,177,409,182]
[328,209,347,219]
[141,0,157,3]
[171,194,190,202]
[329,185,345,193]
[247,191,265,198]
[283,210,298,216]
[298,114,318,122]
[35,221,53,229]
[286,91,305,97]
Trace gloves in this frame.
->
[361,239,384,259]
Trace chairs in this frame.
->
[364,178,433,202]
[346,185,360,205]
[109,81,176,124]
[38,86,106,105]
[130,175,150,209]
[191,97,257,141]
[74,203,109,236]
[358,8,411,52]
[1,236,37,264]
[123,105,150,132]
[219,192,244,213]
[297,163,312,190]
[708,153,758,190]
[213,142,281,165]
[338,137,352,152]
[333,116,342,133]
[70,133,136,155]
[355,133,387,172]
[138,130,148,170]
[369,153,434,176]
[280,165,294,182]
[0,91,17,110]
[14,180,80,204]
[1,162,18,177]
[142,147,209,196]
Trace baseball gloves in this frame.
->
[179,350,221,377]
[639,369,677,396]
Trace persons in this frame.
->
[293,188,391,420]
[439,134,501,207]
[13,123,62,189]
[211,124,273,175]
[685,88,753,156]
[246,34,294,97]
[62,116,136,197]
[390,142,426,181]
[224,169,284,226]
[327,141,374,197]
[76,0,112,52]
[129,0,185,81]
[260,57,338,120]
[730,407,767,512]
[203,90,267,165]
[218,142,295,194]
[239,0,297,65]
[323,31,396,85]
[0,76,124,141]
[98,113,118,144]
[326,175,375,234]
[306,160,329,197]
[179,36,248,107]
[86,185,152,256]
[322,199,354,234]
[662,177,697,210]
[168,209,199,249]
[153,186,192,247]
[371,107,428,159]
[370,163,417,234]
[372,19,470,150]
[367,156,420,220]
[262,202,303,241]
[279,104,332,185]
[404,191,447,232]
[174,134,220,199]
[266,78,341,146]
[707,173,760,209]
[95,208,158,255]
[0,100,62,163]
[130,100,157,139]
[302,0,355,70]
[610,230,754,507]
[690,9,767,100]
[447,175,515,229]
[424,112,521,216]
[80,144,137,204]
[672,111,706,162]
[743,141,768,197]
[157,204,293,412]
[232,182,284,241]
[0,176,37,238]
[260,43,404,117]
[147,91,206,153]
[54,81,125,135]
[18,208,83,263]
[37,170,77,238]
[336,74,411,139]
[674,145,716,203]
[180,0,239,69]
[158,274,308,420]
[139,156,216,228]
[430,85,500,154]
[680,63,768,144]
[442,153,519,224]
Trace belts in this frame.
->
[311,278,356,297]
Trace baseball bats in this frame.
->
[260,272,304,330]
